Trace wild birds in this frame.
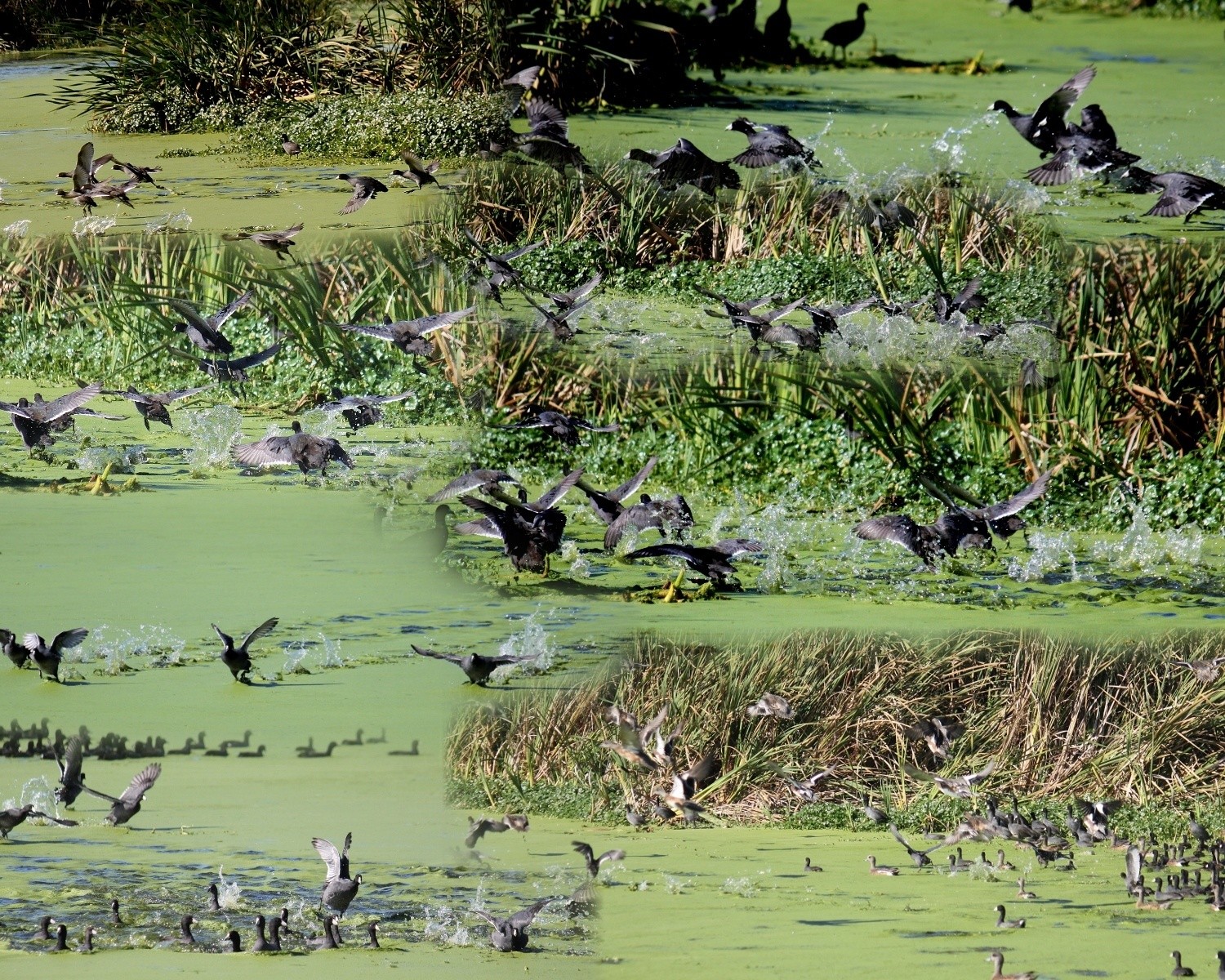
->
[0,1,1225,978]
